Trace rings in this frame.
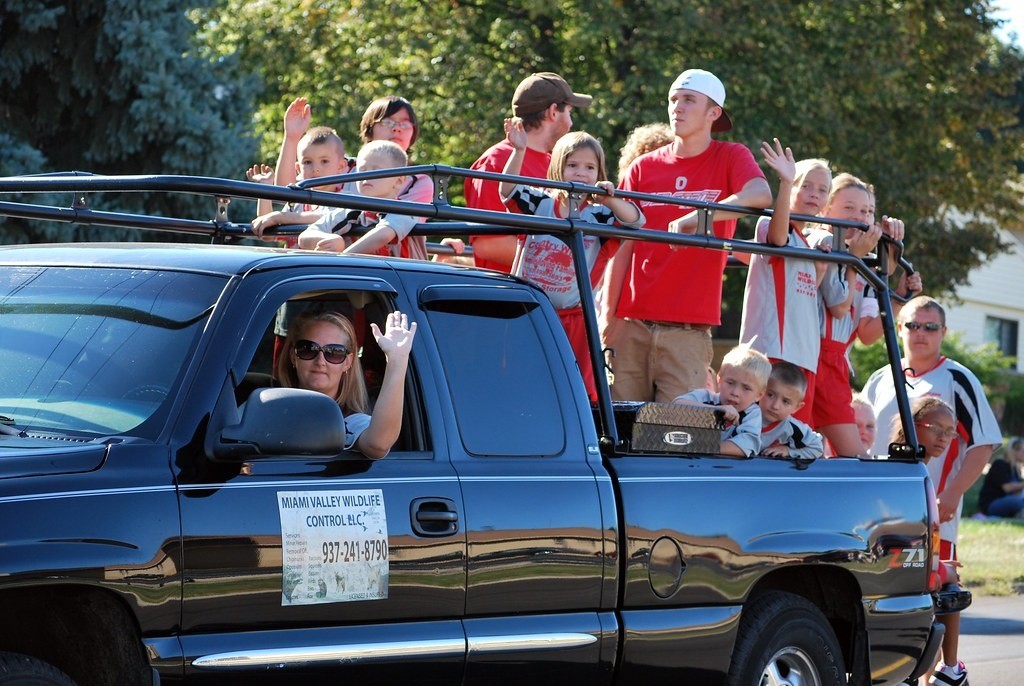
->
[949,514,953,518]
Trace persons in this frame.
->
[463,72,592,272]
[246,96,475,265]
[593,123,1003,686]
[601,68,775,404]
[978,437,1024,518]
[498,118,646,400]
[276,310,418,460]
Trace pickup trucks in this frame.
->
[0,164,974,685]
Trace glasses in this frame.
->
[900,322,944,332]
[293,339,350,364]
[379,117,417,129]
[913,422,960,440]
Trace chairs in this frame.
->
[234,372,279,408]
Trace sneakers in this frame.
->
[929,659,969,686]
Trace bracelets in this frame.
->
[600,194,609,204]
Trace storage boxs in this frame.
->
[592,400,726,455]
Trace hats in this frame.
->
[669,69,733,132]
[511,72,593,116]
